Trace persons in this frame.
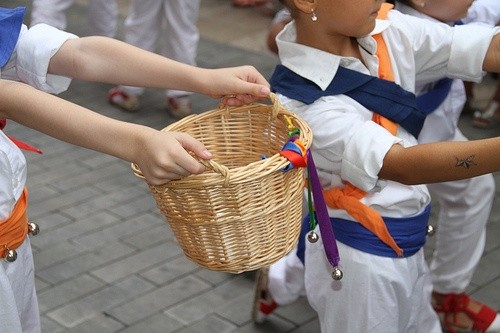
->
[265,0,500,333]
[28,0,118,38]
[0,6,271,333]
[108,0,201,121]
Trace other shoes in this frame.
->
[252,270,278,322]
[473,103,500,127]
[108,88,140,111]
[432,290,499,333]
[164,95,191,117]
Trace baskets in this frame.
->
[130,93,313,274]
